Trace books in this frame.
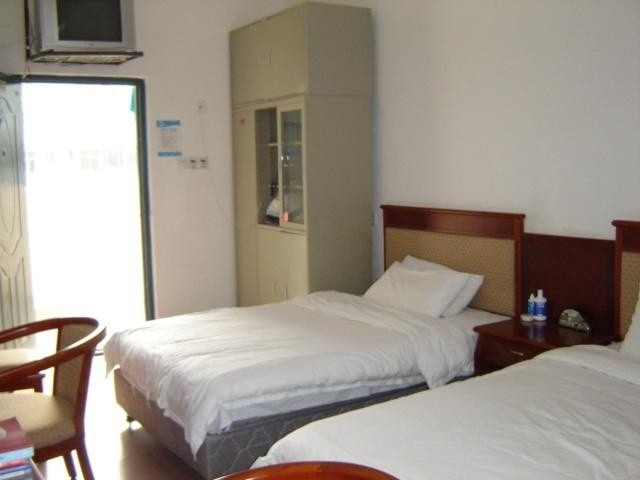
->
[0,417,35,480]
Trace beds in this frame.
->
[248,219,640,480]
[104,204,525,480]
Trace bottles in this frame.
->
[527,289,549,322]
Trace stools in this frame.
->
[0,348,45,392]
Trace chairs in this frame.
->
[214,461,399,480]
[0,317,107,480]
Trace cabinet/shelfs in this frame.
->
[229,2,374,307]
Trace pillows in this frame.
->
[402,254,484,317]
[619,289,640,357]
[364,260,469,318]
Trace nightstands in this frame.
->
[473,316,616,377]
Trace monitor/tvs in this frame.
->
[32,0,136,50]
